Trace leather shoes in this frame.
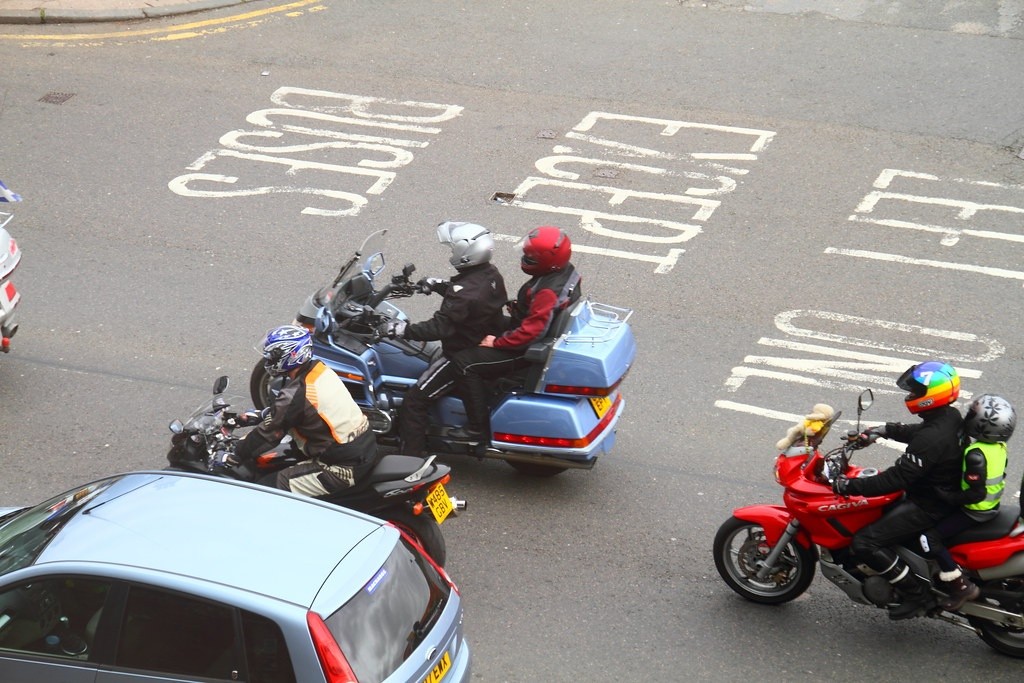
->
[446,424,489,442]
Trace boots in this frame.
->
[877,555,936,620]
[940,564,979,611]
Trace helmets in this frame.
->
[263,324,314,377]
[904,360,961,414]
[520,226,571,277]
[447,223,494,269]
[965,394,1017,442]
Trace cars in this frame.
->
[0,468,475,683]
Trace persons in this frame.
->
[207,325,380,501]
[833,360,972,620]
[372,219,508,456]
[918,392,1017,611]
[446,226,583,442]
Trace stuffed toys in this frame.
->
[777,404,833,451]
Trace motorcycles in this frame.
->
[711,390,1023,660]
[164,377,468,568]
[0,207,22,355]
[247,228,639,478]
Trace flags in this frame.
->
[0,181,22,202]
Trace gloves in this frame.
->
[207,450,235,471]
[417,277,437,295]
[237,407,270,426]
[833,473,850,496]
[870,423,887,437]
[376,314,406,338]
